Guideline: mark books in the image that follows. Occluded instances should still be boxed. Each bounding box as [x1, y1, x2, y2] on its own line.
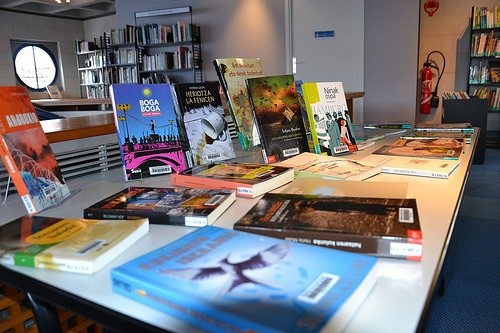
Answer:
[471, 30, 500, 58]
[0, 57, 474, 216]
[143, 45, 199, 71]
[472, 87, 500, 110]
[235, 193, 424, 261]
[76, 38, 100, 53]
[470, 59, 500, 83]
[136, 21, 196, 45]
[91, 50, 111, 66]
[111, 224, 381, 333]
[109, 24, 136, 45]
[472, 6, 500, 30]
[112, 46, 145, 64]
[0, 214, 149, 274]
[84, 186, 236, 228]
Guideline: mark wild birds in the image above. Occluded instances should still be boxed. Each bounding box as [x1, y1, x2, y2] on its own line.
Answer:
[156, 241, 293, 303]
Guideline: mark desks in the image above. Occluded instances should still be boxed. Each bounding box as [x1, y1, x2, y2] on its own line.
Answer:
[0, 126, 481, 333]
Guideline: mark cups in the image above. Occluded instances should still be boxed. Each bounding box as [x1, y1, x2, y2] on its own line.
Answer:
[201, 110, 227, 141]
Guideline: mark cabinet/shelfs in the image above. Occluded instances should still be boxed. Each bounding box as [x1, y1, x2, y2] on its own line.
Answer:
[466, 6, 500, 113]
[74, 24, 203, 112]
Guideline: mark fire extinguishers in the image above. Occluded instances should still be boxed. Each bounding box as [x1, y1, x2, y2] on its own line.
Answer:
[420, 50, 446, 114]
